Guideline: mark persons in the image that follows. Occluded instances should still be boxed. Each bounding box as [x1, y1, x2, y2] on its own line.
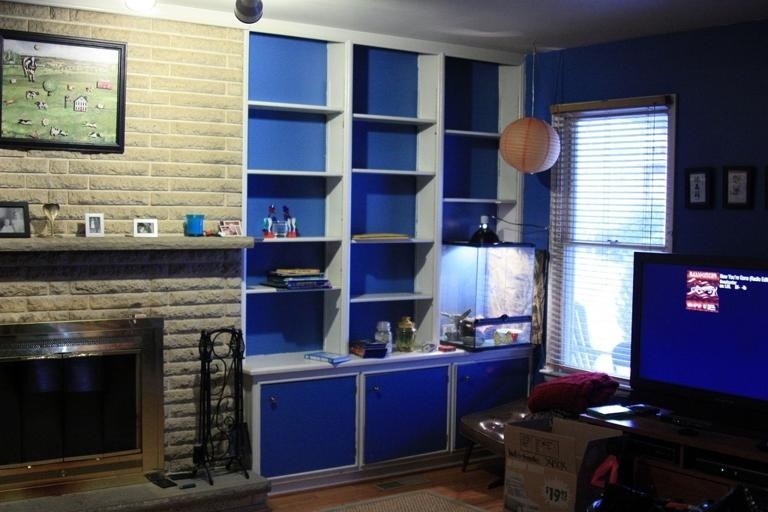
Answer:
[2, 219, 14, 233]
[222, 221, 241, 235]
[90, 217, 101, 233]
[138, 222, 151, 233]
[12, 211, 24, 232]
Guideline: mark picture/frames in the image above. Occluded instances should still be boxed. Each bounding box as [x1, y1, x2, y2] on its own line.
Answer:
[683, 166, 714, 210]
[2, 200, 32, 239]
[132, 218, 161, 239]
[0, 29, 128, 155]
[84, 211, 105, 239]
[721, 165, 755, 210]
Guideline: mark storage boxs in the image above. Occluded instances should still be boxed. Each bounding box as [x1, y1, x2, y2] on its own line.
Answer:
[502, 416, 624, 512]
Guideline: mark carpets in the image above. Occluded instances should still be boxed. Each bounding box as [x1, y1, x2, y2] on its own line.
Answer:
[317, 490, 486, 512]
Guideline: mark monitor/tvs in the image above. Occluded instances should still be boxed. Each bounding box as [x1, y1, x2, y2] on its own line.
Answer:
[630, 252, 768, 435]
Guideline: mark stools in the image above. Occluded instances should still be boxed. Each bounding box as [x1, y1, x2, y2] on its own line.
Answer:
[458, 391, 535, 473]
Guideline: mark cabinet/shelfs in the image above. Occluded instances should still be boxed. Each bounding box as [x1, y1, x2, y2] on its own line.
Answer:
[242, 373, 362, 497]
[361, 361, 452, 483]
[441, 45, 524, 248]
[451, 349, 535, 466]
[347, 36, 443, 355]
[244, 17, 347, 359]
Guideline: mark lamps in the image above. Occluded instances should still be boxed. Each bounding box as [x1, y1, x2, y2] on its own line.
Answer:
[469, 211, 549, 247]
[497, 39, 563, 178]
[233, 0, 266, 24]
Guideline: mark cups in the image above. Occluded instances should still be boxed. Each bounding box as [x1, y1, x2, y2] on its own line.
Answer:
[185, 212, 205, 236]
[271, 220, 289, 237]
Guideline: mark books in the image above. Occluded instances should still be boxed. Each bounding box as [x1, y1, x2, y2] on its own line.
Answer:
[351, 232, 408, 238]
[259, 268, 332, 290]
[303, 352, 350, 364]
[352, 236, 409, 241]
[349, 338, 386, 358]
[587, 404, 632, 420]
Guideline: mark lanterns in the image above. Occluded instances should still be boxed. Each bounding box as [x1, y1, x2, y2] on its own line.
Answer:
[498, 115, 562, 175]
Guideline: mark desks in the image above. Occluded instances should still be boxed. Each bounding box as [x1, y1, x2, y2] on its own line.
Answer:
[577, 412, 768, 506]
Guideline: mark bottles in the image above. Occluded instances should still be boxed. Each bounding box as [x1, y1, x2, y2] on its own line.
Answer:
[375, 320, 392, 353]
[396, 314, 415, 352]
